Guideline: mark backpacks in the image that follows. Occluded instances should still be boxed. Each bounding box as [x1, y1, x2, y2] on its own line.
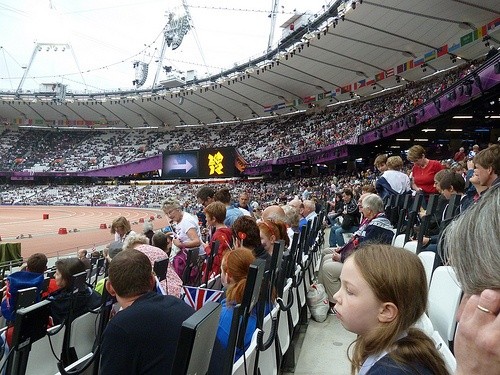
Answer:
[173, 241, 200, 283]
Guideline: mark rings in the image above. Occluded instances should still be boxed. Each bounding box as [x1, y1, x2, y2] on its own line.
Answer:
[477, 305, 496, 315]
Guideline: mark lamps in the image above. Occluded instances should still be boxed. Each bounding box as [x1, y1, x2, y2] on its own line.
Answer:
[449, 54, 457, 64]
[482, 35, 492, 49]
[2, 0, 377, 131]
[395, 75, 401, 83]
[420, 63, 428, 73]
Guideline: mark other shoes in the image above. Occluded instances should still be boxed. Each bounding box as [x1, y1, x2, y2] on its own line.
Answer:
[327, 306, 337, 315]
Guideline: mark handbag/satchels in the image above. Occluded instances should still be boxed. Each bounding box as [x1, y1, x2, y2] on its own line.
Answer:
[342, 215, 354, 230]
[309, 282, 329, 322]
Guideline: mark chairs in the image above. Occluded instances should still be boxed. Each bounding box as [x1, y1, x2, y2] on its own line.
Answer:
[0, 190, 500, 375]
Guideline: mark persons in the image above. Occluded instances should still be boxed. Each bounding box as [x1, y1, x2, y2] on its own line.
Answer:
[444, 182, 500, 375]
[333, 244, 449, 375]
[0, 47, 500, 375]
[213, 248, 274, 375]
[99, 249, 196, 375]
